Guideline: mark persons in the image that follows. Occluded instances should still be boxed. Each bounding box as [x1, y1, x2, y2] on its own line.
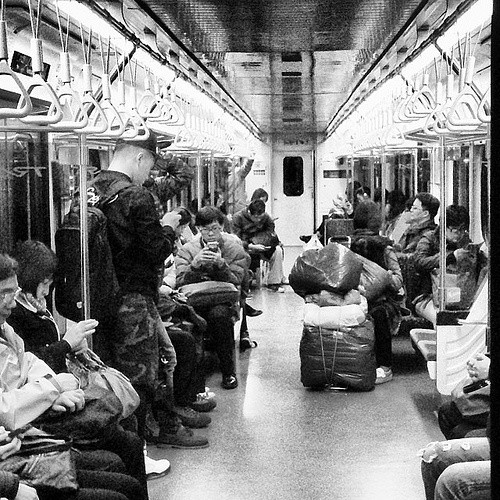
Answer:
[159, 152, 288, 425]
[420, 354, 491, 500]
[299, 180, 479, 385]
[0, 239, 148, 500]
[70, 124, 208, 479]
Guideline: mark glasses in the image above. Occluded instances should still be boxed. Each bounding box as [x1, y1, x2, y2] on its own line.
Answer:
[1, 286, 23, 303]
[199, 224, 218, 236]
[448, 228, 468, 234]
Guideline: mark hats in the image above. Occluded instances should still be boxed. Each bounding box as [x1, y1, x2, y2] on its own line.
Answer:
[173, 206, 192, 225]
[116, 127, 161, 161]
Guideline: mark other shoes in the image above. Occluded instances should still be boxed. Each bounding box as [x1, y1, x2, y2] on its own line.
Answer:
[299, 236, 311, 243]
[172, 406, 213, 428]
[156, 417, 210, 449]
[374, 368, 393, 385]
[188, 394, 217, 413]
[241, 331, 252, 348]
[145, 455, 171, 481]
[221, 366, 238, 389]
[266, 284, 286, 292]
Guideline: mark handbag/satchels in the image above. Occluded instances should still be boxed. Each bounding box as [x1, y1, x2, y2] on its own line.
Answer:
[32, 385, 124, 439]
[451, 374, 490, 416]
[0, 423, 79, 495]
[178, 280, 239, 310]
[430, 255, 477, 309]
[74, 350, 141, 422]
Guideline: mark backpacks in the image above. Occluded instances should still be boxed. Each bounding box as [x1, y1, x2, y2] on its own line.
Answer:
[54, 180, 138, 332]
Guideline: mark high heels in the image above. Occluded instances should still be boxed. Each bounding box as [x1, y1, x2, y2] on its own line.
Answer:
[246, 309, 263, 316]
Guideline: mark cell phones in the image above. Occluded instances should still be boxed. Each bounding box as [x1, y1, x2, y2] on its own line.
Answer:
[207, 242, 219, 253]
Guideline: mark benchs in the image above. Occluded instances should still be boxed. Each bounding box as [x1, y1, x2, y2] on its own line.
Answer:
[409, 271, 490, 363]
